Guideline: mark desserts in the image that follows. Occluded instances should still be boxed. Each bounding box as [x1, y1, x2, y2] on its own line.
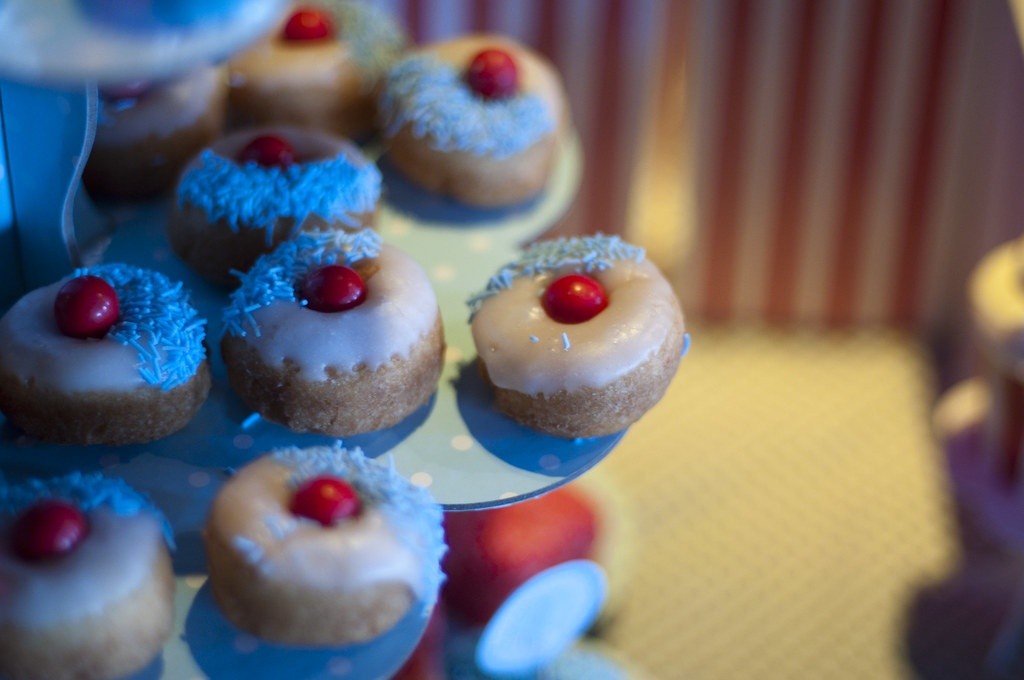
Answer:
[0, 0, 685, 680]
